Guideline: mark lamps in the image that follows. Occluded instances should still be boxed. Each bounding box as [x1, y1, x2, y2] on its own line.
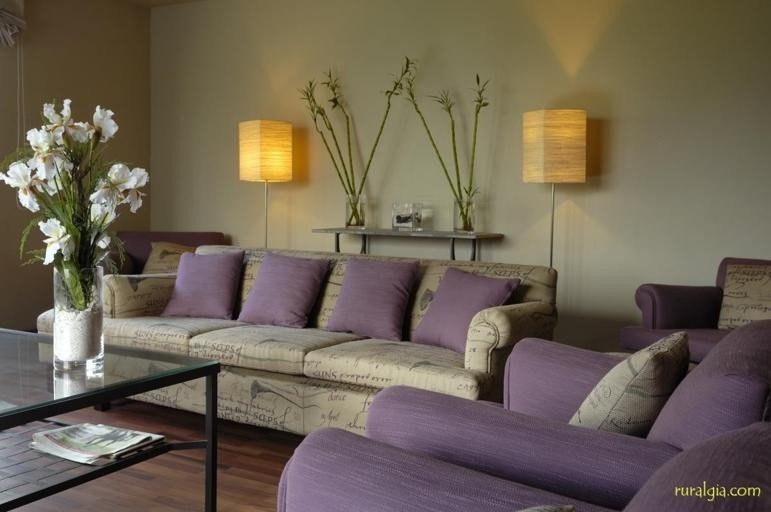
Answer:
[238, 119, 292, 248]
[521, 109, 588, 268]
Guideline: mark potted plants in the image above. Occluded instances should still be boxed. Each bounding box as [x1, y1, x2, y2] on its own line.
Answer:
[295, 55, 415, 230]
[400, 70, 491, 233]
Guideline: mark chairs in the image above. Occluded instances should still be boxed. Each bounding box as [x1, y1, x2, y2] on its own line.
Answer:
[97, 231, 223, 275]
[618, 257, 771, 364]
[365, 318, 771, 512]
[276, 422, 771, 512]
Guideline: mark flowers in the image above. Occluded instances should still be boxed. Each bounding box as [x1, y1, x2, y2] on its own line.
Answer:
[0, 97, 150, 310]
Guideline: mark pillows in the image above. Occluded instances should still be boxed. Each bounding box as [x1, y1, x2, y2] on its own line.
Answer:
[160, 250, 246, 320]
[568, 330, 690, 436]
[142, 241, 197, 275]
[238, 251, 337, 328]
[324, 258, 420, 341]
[413, 267, 521, 356]
[717, 264, 771, 330]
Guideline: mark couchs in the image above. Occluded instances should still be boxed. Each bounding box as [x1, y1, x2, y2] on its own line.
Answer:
[37, 244, 557, 436]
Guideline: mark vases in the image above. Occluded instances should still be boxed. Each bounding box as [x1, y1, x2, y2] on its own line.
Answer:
[53, 266, 104, 370]
[52, 369, 104, 399]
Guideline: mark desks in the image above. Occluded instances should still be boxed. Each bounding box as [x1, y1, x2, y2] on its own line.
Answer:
[311, 227, 505, 262]
